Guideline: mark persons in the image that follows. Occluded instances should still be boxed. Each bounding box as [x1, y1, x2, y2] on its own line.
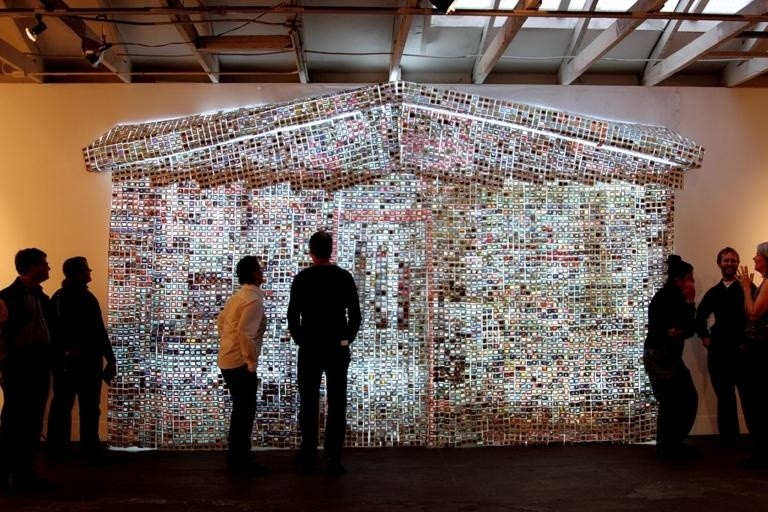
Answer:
[288, 231, 361, 478]
[0, 249, 53, 496]
[218, 257, 267, 475]
[644, 242, 768, 468]
[43, 257, 116, 466]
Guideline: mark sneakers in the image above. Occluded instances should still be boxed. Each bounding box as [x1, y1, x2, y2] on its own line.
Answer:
[294, 457, 348, 478]
[225, 447, 266, 472]
[43, 439, 118, 461]
[719, 433, 768, 468]
[1, 471, 60, 498]
[654, 441, 703, 461]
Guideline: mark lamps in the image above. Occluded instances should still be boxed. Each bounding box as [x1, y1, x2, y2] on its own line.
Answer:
[22, 13, 48, 42]
[79, 35, 112, 67]
